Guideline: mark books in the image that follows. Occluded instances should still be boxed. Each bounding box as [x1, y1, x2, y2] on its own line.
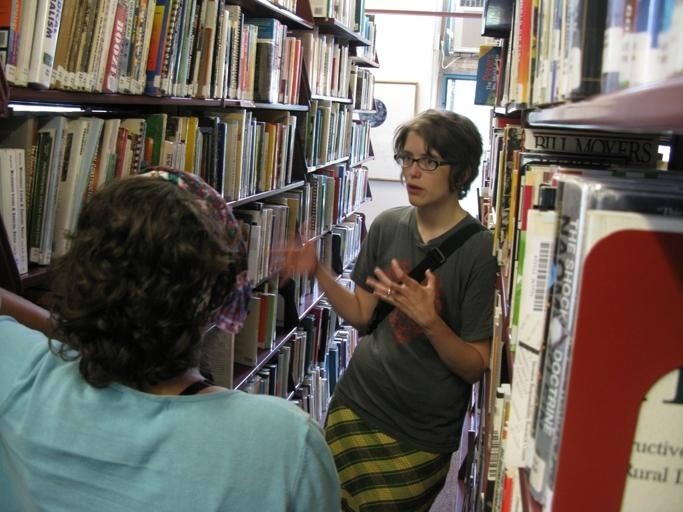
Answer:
[465, 2, 682, 511]
[1, 1, 375, 422]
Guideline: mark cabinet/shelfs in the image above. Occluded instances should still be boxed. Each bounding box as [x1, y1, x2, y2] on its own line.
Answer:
[470, 1, 682, 512]
[0, 1, 377, 429]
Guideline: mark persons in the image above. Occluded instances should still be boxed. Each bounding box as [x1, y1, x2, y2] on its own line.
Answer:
[0, 167, 341, 512]
[269, 108, 497, 512]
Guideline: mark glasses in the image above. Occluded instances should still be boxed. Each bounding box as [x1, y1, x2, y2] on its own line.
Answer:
[394, 153, 452, 171]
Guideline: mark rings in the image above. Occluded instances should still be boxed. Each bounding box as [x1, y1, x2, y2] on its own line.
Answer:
[384, 287, 392, 296]
